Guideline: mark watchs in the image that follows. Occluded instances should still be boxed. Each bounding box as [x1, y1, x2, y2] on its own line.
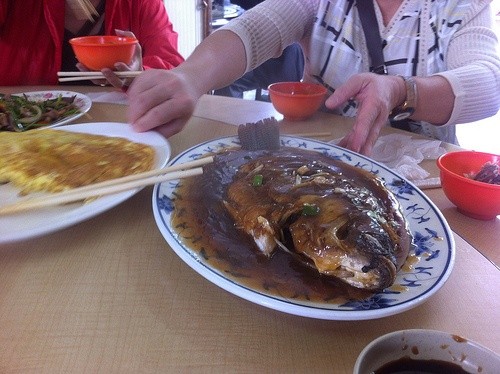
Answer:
[388, 74, 418, 121]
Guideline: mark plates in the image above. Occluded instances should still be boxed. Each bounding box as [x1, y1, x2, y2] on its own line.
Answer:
[11, 89, 92, 130]
[0, 122, 170, 244]
[151, 138, 457, 321]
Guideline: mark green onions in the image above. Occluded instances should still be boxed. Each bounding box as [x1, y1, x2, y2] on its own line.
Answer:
[252, 165, 350, 215]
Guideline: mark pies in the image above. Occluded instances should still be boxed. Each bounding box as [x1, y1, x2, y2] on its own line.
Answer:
[0, 130, 157, 205]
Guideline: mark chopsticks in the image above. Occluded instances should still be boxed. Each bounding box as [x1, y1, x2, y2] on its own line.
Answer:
[57, 71, 146, 82]
[0, 154, 213, 216]
[78, 0, 99, 24]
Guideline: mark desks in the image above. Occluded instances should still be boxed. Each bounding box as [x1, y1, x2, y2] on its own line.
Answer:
[0, 85, 500, 374]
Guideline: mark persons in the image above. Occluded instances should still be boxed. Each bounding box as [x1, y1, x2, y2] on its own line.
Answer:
[126, 0, 500, 140]
[214, 0, 305, 98]
[0, 0, 185, 88]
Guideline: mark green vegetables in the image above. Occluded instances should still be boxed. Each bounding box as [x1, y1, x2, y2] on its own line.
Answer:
[0, 95, 80, 132]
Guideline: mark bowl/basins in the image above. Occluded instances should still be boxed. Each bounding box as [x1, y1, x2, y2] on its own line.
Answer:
[70, 35, 138, 72]
[267, 82, 328, 121]
[436, 150, 500, 221]
[352, 328, 500, 374]
